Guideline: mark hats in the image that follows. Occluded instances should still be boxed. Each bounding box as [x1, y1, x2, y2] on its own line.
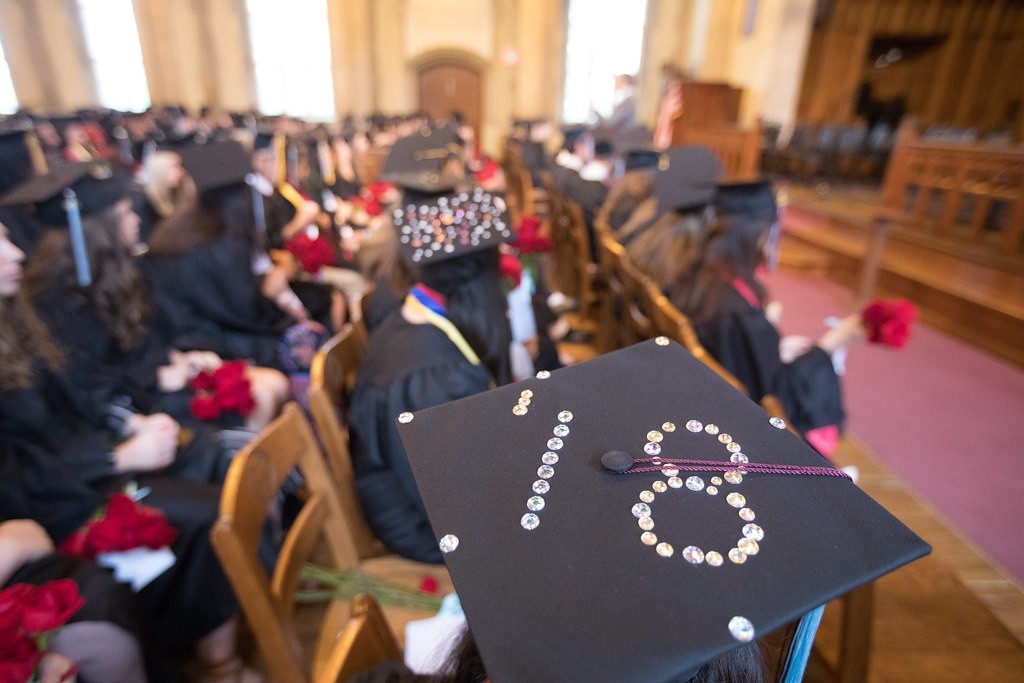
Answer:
[393, 335, 932, 683]
[0, 92, 788, 280]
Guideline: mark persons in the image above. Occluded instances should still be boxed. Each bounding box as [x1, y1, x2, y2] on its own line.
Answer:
[514, 73, 868, 486]
[0, 103, 567, 683]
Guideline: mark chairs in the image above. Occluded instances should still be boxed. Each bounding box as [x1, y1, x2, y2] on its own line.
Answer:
[503, 145, 749, 398]
[208, 293, 456, 683]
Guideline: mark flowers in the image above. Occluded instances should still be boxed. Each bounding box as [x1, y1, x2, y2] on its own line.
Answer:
[351, 181, 390, 217]
[499, 251, 523, 292]
[514, 216, 555, 255]
[191, 358, 255, 420]
[477, 155, 500, 184]
[287, 232, 336, 274]
[63, 489, 180, 556]
[0, 579, 85, 683]
[854, 298, 919, 350]
